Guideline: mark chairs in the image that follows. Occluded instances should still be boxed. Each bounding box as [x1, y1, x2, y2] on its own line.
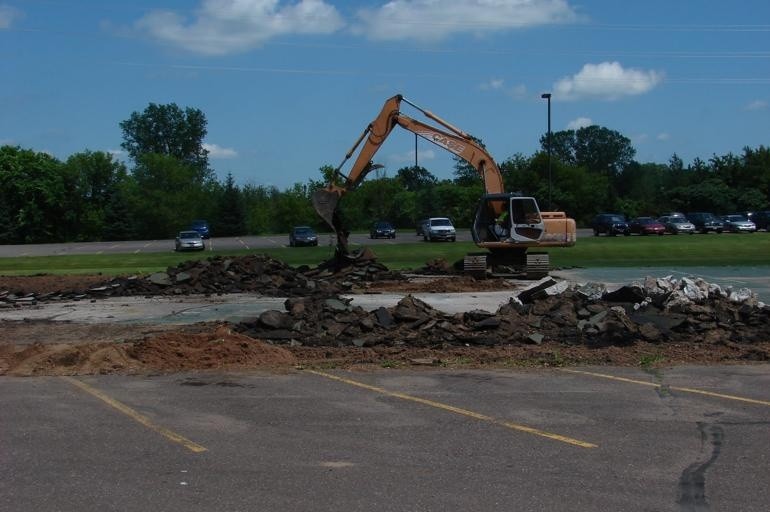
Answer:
[493, 204, 511, 242]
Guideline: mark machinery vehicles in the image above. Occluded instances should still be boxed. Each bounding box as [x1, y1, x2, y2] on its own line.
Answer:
[311, 93, 578, 280]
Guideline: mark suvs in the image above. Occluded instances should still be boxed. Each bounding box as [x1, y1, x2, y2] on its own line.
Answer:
[750, 207, 770, 232]
[686, 212, 725, 234]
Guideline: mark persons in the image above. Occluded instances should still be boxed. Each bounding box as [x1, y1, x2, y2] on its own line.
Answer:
[489, 202, 509, 241]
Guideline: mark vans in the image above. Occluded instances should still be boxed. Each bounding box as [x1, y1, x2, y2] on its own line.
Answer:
[191, 219, 211, 239]
[422, 217, 456, 242]
[593, 212, 633, 237]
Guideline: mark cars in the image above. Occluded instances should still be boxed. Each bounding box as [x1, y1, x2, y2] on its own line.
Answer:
[416, 218, 429, 235]
[657, 215, 696, 235]
[175, 230, 206, 252]
[663, 213, 688, 222]
[369, 220, 396, 239]
[289, 226, 319, 247]
[722, 214, 757, 233]
[628, 216, 666, 237]
